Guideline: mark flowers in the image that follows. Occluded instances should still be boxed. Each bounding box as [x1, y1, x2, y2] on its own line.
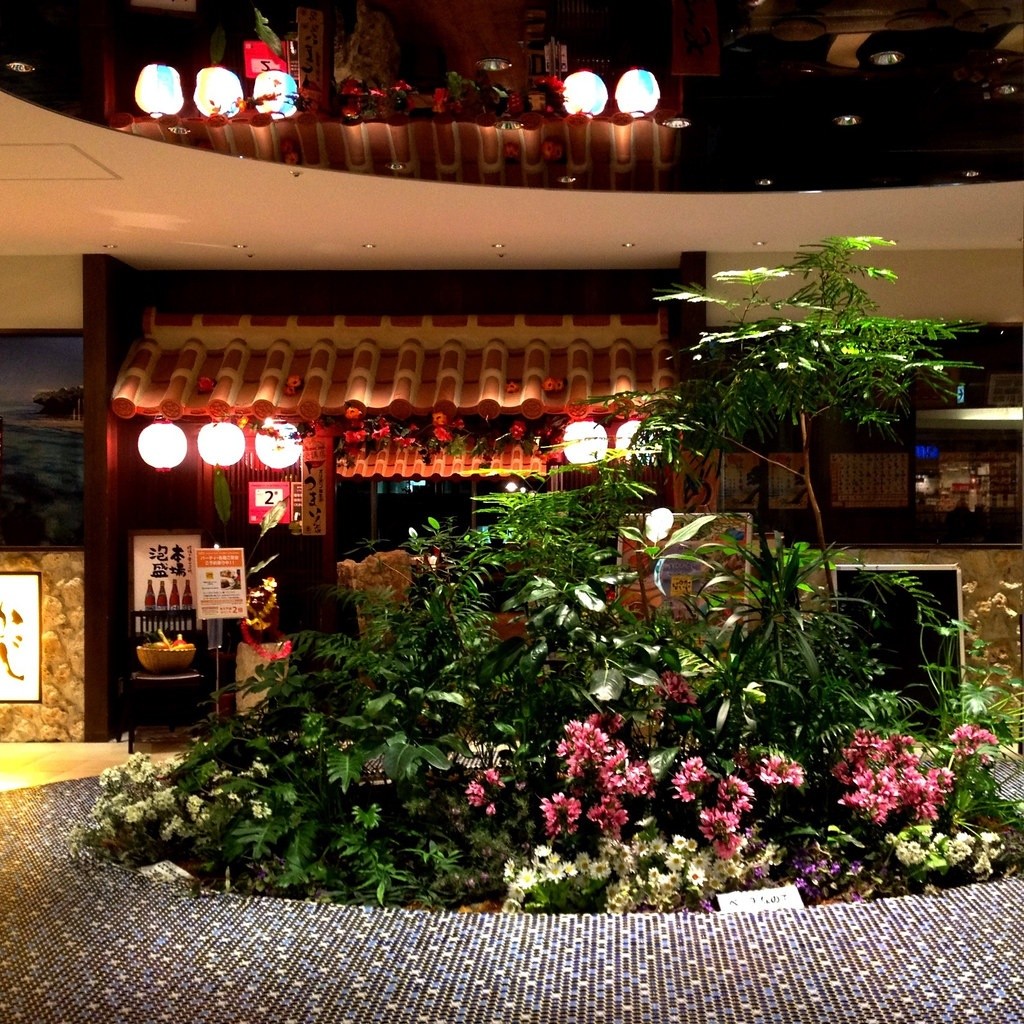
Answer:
[312, 407, 544, 462]
[332, 73, 568, 119]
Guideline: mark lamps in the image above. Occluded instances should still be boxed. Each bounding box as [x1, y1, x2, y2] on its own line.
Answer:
[197, 422, 246, 471]
[192, 66, 244, 121]
[561, 71, 608, 119]
[255, 422, 302, 474]
[252, 70, 300, 121]
[560, 420, 608, 465]
[618, 69, 661, 119]
[617, 419, 657, 466]
[136, 414, 188, 474]
[134, 63, 184, 119]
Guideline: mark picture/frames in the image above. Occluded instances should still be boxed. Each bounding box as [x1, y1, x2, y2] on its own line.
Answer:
[830, 562, 966, 744]
[610, 512, 753, 642]
[0, 328, 84, 551]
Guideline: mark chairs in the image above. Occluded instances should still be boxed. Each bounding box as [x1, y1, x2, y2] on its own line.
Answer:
[115, 609, 207, 755]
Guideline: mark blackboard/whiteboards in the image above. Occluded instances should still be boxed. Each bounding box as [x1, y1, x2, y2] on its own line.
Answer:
[828, 562, 966, 739]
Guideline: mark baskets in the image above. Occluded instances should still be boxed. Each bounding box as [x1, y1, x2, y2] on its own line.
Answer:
[137, 645, 196, 674]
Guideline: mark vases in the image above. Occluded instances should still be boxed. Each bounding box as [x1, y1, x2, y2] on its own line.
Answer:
[528, 91, 547, 110]
